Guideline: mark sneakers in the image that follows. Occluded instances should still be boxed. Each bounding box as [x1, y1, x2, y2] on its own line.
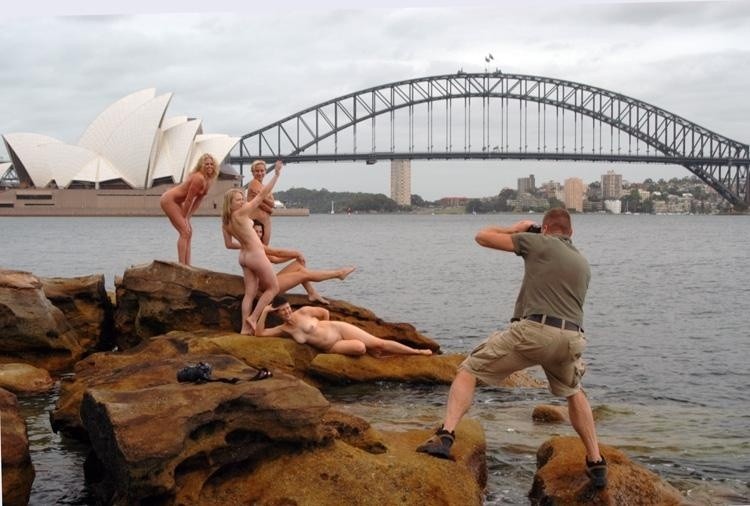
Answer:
[585, 454, 608, 488]
[415, 424, 455, 456]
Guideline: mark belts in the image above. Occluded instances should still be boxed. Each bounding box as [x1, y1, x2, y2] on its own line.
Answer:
[510, 313, 586, 335]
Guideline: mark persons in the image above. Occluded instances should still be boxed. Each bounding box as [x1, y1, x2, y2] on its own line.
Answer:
[251, 219, 356, 305]
[160, 153, 219, 265]
[247, 160, 276, 246]
[221, 160, 282, 335]
[416, 210, 608, 487]
[254, 296, 433, 358]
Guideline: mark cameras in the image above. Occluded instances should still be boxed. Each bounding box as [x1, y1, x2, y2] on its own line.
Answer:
[177, 363, 209, 384]
[527, 224, 543, 235]
